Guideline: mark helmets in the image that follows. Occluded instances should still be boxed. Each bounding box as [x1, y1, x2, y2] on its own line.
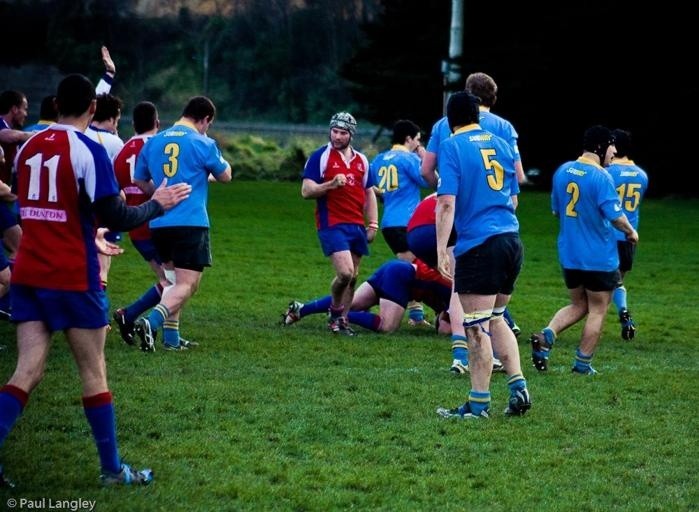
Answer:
[583, 125, 617, 167]
[447, 91, 479, 134]
[585, 127, 615, 167]
[329, 111, 358, 142]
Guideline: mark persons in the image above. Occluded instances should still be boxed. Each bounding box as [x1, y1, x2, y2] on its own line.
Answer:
[1, 72, 154, 491]
[602, 126, 651, 342]
[530, 120, 639, 378]
[282, 71, 532, 421]
[1, 44, 234, 354]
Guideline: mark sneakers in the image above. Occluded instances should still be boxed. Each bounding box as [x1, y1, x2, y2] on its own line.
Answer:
[282, 301, 635, 415]
[112, 306, 200, 353]
[99, 464, 155, 498]
